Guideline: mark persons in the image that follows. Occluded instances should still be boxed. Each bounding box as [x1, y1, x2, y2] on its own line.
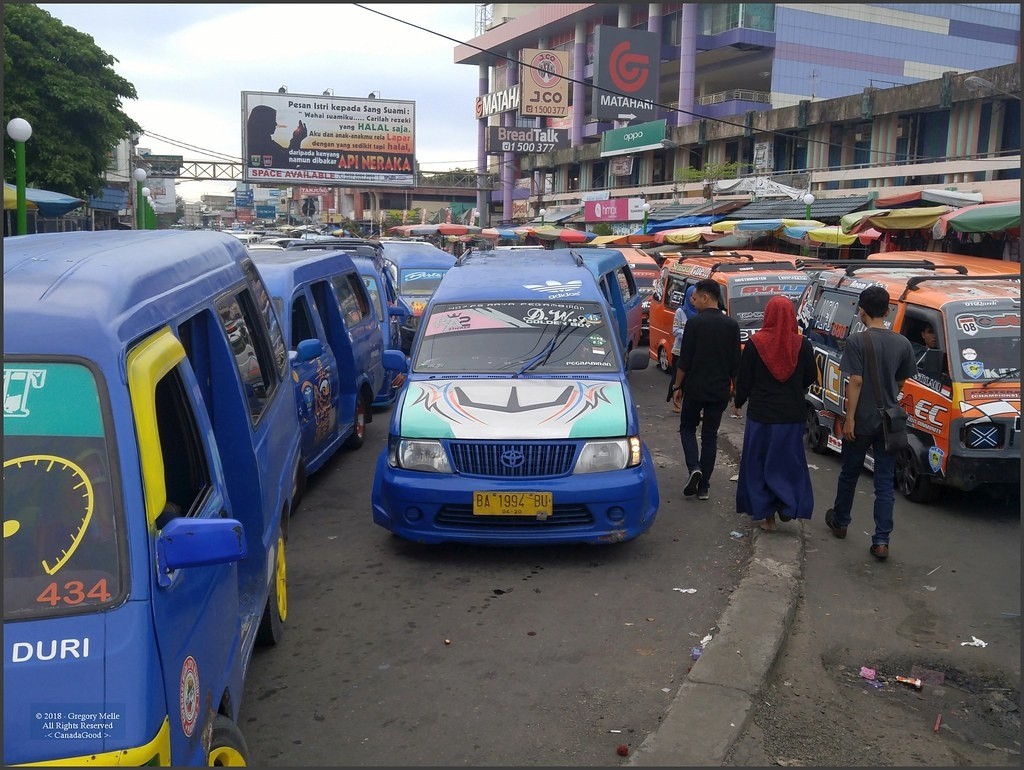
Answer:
[823, 285, 918, 558]
[734, 296, 818, 531]
[912, 323, 951, 368]
[667, 278, 742, 500]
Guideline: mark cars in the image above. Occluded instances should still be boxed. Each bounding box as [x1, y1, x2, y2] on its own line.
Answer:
[373, 248, 660, 548]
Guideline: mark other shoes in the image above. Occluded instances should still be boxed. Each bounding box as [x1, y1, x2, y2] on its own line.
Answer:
[673, 407, 680, 412]
[826, 509, 848, 538]
[870, 545, 888, 557]
[760, 519, 776, 530]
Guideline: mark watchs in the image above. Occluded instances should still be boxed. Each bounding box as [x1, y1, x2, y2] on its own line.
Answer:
[672, 385, 681, 390]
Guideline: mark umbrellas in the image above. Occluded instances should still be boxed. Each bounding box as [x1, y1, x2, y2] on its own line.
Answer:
[389, 220, 481, 253]
[483, 216, 596, 252]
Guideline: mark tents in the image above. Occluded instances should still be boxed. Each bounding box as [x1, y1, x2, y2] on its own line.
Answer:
[2, 184, 87, 237]
[570, 212, 863, 261]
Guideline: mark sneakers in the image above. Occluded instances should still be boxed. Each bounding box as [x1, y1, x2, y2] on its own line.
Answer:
[697, 483, 710, 499]
[683, 468, 702, 496]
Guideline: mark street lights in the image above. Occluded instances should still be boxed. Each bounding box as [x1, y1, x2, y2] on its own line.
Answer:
[804, 194, 815, 221]
[643, 202, 651, 235]
[539, 208, 547, 227]
[474, 212, 482, 228]
[134, 166, 147, 230]
[7, 117, 32, 234]
[141, 187, 150, 229]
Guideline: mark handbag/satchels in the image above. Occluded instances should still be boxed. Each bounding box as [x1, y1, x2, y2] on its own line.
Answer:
[878, 407, 909, 452]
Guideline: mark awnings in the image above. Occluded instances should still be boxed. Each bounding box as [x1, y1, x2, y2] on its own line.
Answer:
[640, 189, 1022, 218]
[531, 208, 585, 223]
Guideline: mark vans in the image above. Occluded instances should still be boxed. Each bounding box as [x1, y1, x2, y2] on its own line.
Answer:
[3, 227, 303, 769]
[784, 256, 1021, 505]
[211, 250, 386, 521]
[222, 219, 1021, 380]
